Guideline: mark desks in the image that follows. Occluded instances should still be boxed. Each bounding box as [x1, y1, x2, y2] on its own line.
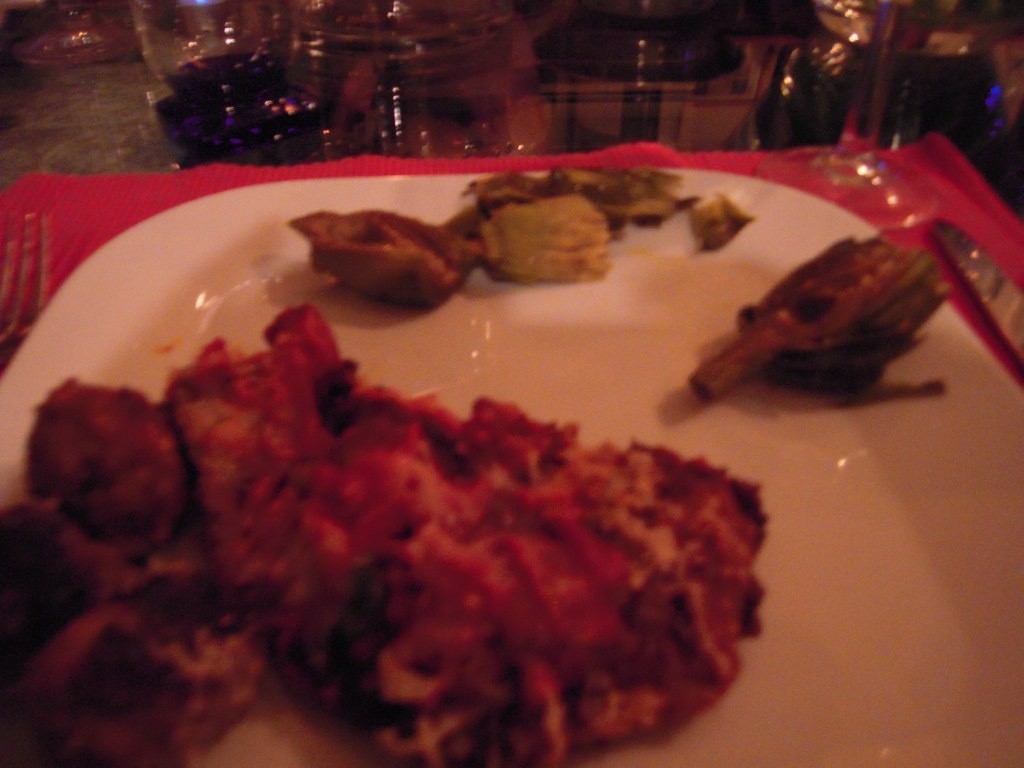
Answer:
[1, 137, 1024, 419]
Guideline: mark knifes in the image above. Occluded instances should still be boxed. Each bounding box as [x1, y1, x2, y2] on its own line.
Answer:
[933, 216, 1024, 366]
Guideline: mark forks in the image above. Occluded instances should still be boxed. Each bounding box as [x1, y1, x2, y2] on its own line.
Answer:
[0, 214, 48, 358]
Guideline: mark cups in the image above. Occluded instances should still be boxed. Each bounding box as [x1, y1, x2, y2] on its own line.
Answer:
[1, 0, 1024, 165]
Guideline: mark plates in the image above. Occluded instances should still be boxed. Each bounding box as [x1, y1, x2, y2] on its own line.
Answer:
[3, 170, 1024, 767]
